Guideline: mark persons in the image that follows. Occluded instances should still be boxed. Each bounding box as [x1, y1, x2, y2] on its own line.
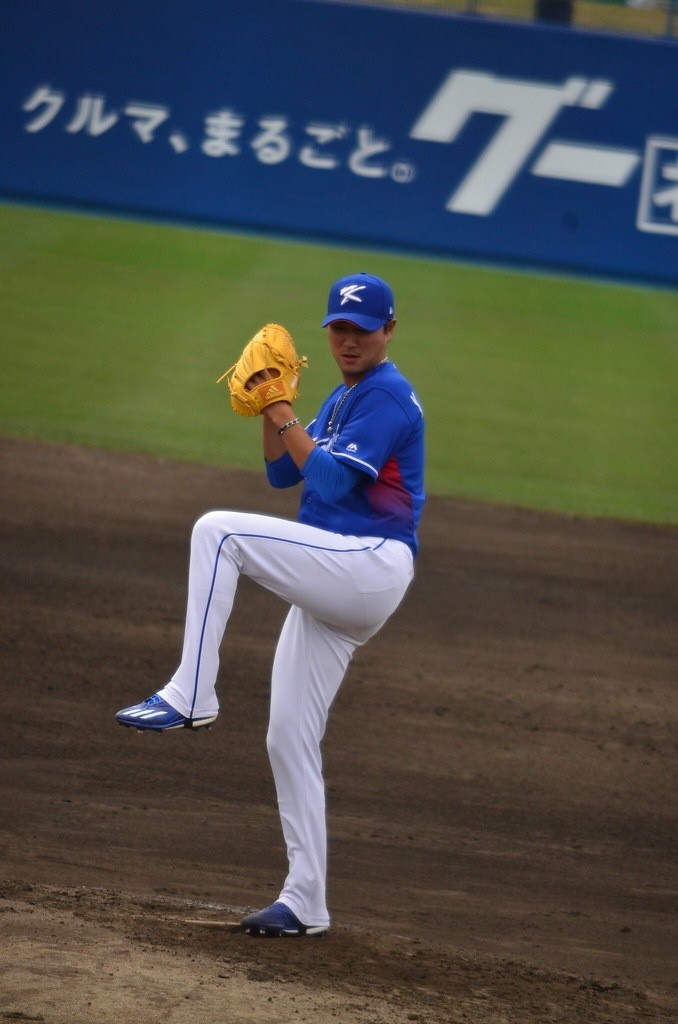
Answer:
[113, 272, 427, 940]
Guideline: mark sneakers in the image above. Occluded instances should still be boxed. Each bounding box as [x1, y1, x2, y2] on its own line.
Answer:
[114, 685, 217, 736]
[240, 902, 329, 939]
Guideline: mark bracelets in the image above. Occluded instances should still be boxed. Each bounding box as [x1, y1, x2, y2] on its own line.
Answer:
[276, 417, 302, 437]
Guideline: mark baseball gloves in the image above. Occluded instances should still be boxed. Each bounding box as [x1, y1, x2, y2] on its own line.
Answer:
[230, 323, 303, 418]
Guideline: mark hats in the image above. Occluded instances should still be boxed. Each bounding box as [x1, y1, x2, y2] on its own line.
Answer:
[321, 271, 395, 331]
